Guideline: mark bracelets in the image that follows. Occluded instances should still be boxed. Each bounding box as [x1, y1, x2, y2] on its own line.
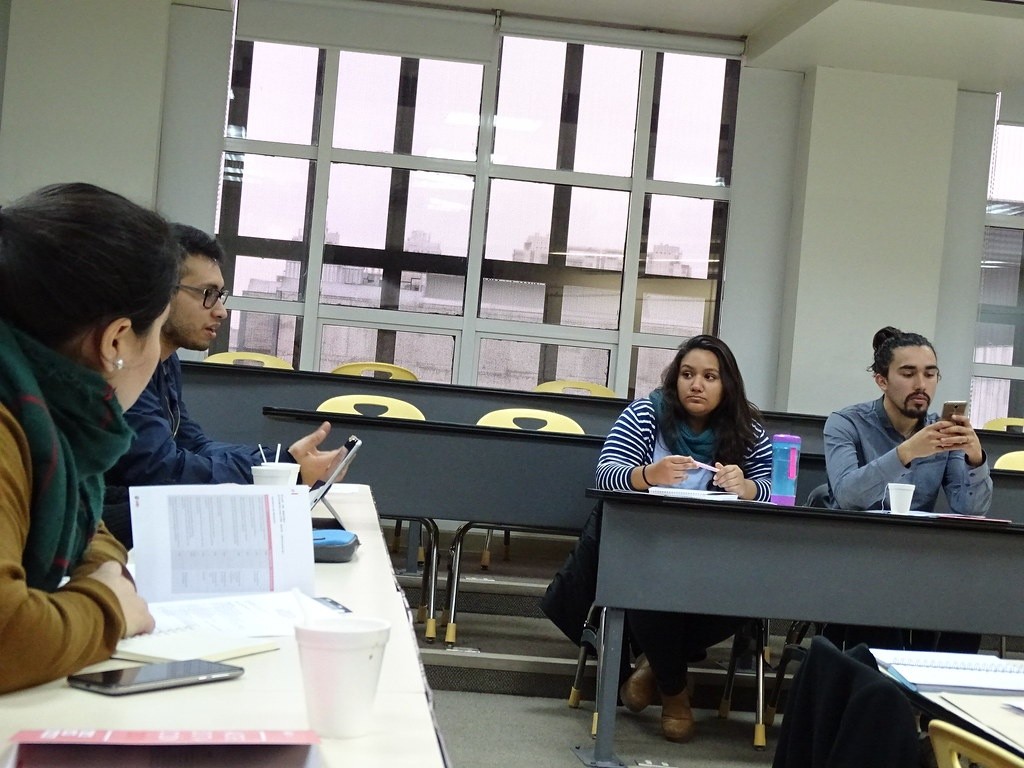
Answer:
[642, 465, 658, 486]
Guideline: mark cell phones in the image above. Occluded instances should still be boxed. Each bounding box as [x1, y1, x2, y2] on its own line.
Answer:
[309, 435, 362, 508]
[942, 401, 967, 434]
[68, 660, 244, 696]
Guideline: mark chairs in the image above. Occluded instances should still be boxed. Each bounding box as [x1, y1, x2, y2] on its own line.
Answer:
[772, 635, 917, 768]
[983, 418, 1024, 431]
[203, 352, 295, 370]
[331, 362, 425, 565]
[316, 395, 440, 644]
[993, 450, 1024, 471]
[480, 380, 616, 568]
[928, 718, 1024, 768]
[446, 408, 585, 645]
[764, 484, 1007, 725]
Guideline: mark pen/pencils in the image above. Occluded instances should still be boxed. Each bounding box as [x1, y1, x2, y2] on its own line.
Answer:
[695, 461, 720, 473]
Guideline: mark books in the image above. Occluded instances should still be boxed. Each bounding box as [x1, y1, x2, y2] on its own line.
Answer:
[869, 648, 1024, 715]
[649, 487, 738, 500]
[111, 485, 340, 662]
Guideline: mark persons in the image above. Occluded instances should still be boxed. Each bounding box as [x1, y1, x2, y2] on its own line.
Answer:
[0, 181, 190, 693]
[595, 334, 773, 743]
[822, 324, 993, 669]
[102, 222, 349, 550]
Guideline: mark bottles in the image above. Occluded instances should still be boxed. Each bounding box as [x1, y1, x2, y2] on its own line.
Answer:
[772, 434, 800, 505]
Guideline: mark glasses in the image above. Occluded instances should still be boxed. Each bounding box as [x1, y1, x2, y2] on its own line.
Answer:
[175, 284, 229, 309]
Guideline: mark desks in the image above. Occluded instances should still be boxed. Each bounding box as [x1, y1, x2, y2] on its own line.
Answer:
[0, 359, 1024, 768]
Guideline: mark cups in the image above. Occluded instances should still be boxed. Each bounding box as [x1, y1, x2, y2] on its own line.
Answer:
[887, 482, 916, 514]
[295, 618, 393, 741]
[263, 463, 300, 485]
[252, 465, 289, 490]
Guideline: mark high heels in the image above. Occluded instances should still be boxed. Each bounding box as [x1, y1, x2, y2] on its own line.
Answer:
[620, 652, 658, 712]
[662, 677, 694, 743]
